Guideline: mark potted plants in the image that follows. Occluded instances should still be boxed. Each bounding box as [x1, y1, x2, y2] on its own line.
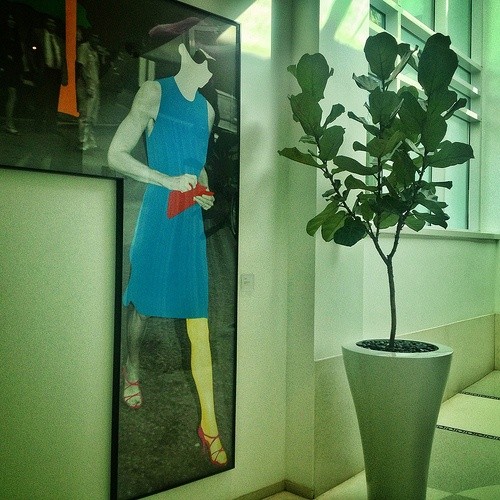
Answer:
[277, 32, 477, 500]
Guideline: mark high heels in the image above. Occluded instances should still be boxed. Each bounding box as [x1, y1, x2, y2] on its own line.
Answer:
[122, 364, 142, 410]
[196, 424, 230, 470]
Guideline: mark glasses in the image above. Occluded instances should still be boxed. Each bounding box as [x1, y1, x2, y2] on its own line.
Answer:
[190, 45, 218, 75]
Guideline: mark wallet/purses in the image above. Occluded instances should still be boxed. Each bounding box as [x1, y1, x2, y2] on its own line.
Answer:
[168, 181, 206, 217]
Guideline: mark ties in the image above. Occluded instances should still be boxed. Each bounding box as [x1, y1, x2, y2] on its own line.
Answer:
[48, 35, 58, 72]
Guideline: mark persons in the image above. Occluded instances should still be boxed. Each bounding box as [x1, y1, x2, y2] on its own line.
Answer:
[105, 20, 230, 468]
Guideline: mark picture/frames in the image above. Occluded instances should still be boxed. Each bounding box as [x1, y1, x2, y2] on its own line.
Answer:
[0, 0, 243, 500]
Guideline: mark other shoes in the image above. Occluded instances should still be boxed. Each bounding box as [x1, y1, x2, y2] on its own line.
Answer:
[5, 125, 18, 135]
[79, 140, 98, 151]
